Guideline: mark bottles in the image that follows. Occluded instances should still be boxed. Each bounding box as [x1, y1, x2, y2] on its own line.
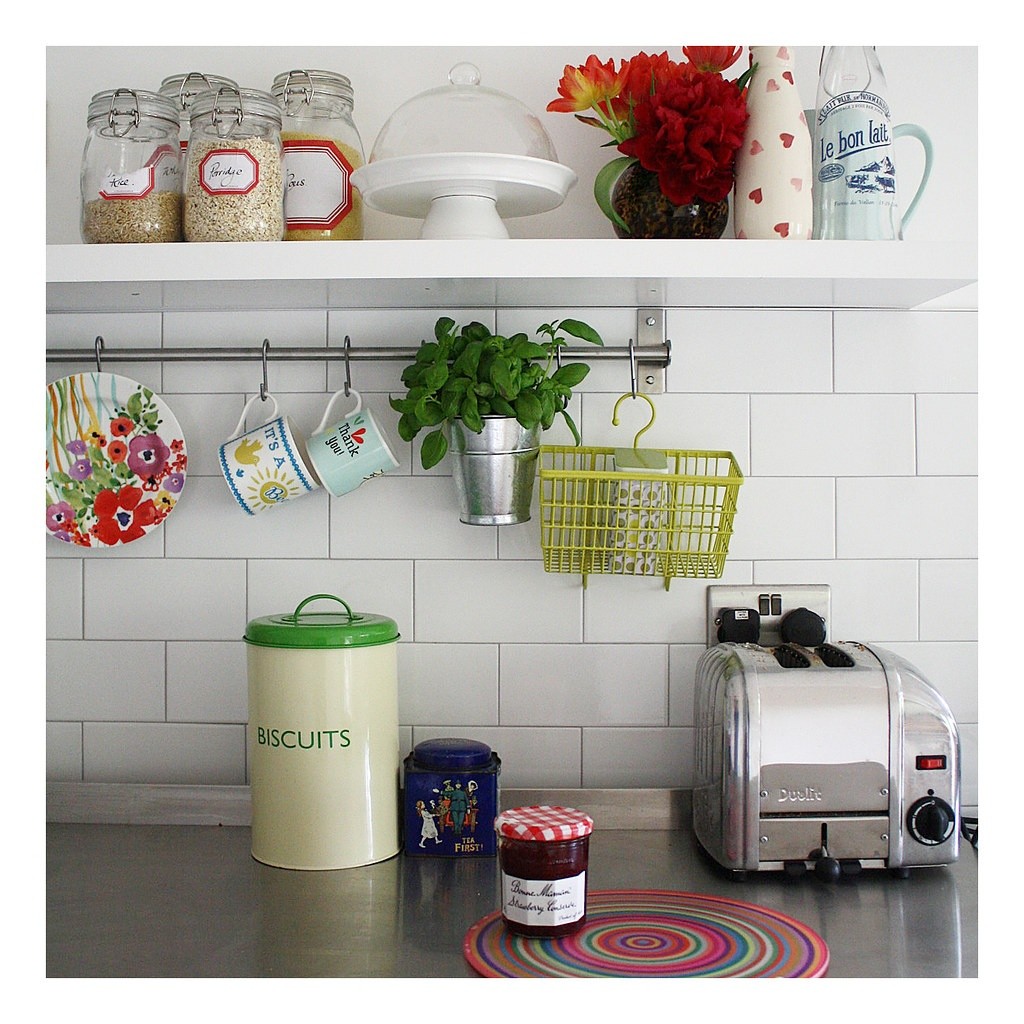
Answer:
[181, 84, 288, 243]
[156, 72, 238, 174]
[732, 46, 817, 238]
[808, 46, 933, 241]
[270, 69, 368, 242]
[78, 90, 186, 244]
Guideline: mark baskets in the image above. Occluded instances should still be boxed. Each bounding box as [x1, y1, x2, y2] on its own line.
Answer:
[540, 393, 743, 592]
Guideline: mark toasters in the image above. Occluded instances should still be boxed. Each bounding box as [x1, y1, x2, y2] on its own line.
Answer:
[694, 636, 964, 878]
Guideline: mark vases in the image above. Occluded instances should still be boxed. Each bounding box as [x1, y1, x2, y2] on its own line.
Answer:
[593, 156, 730, 240]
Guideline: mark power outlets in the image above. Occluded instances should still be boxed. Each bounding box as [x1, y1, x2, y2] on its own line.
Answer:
[707, 583, 832, 648]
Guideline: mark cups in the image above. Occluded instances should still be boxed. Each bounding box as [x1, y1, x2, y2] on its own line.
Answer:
[305, 389, 401, 497]
[218, 393, 320, 516]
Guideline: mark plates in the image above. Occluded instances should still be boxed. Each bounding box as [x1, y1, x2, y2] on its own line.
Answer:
[46, 371, 187, 549]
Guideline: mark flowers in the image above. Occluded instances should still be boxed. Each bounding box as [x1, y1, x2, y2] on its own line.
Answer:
[547, 45, 757, 206]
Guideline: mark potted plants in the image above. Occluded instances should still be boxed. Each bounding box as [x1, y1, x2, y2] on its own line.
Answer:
[387, 316, 604, 526]
